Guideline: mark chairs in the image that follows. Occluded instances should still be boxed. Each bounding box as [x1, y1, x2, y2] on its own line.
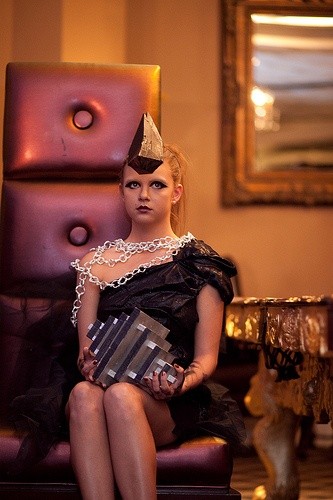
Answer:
[0, 60, 240, 500]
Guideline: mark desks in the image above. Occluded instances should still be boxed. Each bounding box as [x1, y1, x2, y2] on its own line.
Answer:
[227, 295, 333, 499]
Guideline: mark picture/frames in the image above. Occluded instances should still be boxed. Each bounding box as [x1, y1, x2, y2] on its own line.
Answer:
[218, 0, 333, 209]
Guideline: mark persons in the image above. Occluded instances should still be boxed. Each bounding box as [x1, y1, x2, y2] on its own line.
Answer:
[6, 109, 247, 500]
